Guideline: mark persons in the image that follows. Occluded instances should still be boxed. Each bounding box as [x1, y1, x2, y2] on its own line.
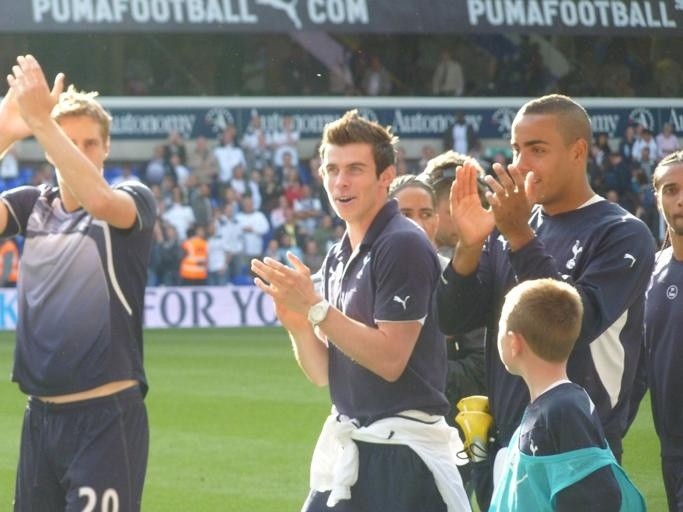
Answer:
[0, 53, 157, 512]
[420, 149, 496, 512]
[489, 277, 646, 512]
[588, 117, 683, 249]
[113, 123, 343, 287]
[250, 109, 474, 512]
[430, 93, 656, 512]
[619, 149, 683, 512]
[385, 174, 493, 512]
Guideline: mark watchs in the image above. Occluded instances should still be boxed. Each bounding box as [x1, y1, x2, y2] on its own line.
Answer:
[307, 298, 330, 327]
